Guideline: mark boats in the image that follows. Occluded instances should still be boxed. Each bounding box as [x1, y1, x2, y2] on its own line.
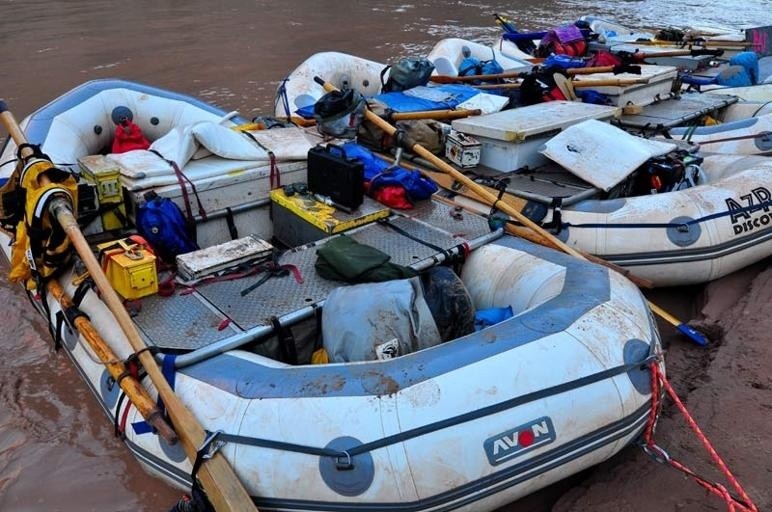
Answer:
[422, 15, 772, 157]
[0, 79, 666, 511]
[273, 51, 772, 287]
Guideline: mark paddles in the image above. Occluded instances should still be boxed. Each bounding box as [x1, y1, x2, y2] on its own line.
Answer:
[624, 26, 772, 55]
[229, 109, 480, 132]
[0, 99, 258, 510]
[373, 151, 547, 223]
[427, 66, 614, 83]
[315, 76, 709, 346]
[553, 71, 578, 102]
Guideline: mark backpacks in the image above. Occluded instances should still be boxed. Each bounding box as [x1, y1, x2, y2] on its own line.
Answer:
[132, 189, 201, 268]
[312, 86, 369, 141]
[378, 56, 436, 95]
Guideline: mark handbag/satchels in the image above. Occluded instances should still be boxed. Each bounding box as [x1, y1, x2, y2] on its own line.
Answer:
[456, 56, 507, 90]
[537, 20, 594, 59]
